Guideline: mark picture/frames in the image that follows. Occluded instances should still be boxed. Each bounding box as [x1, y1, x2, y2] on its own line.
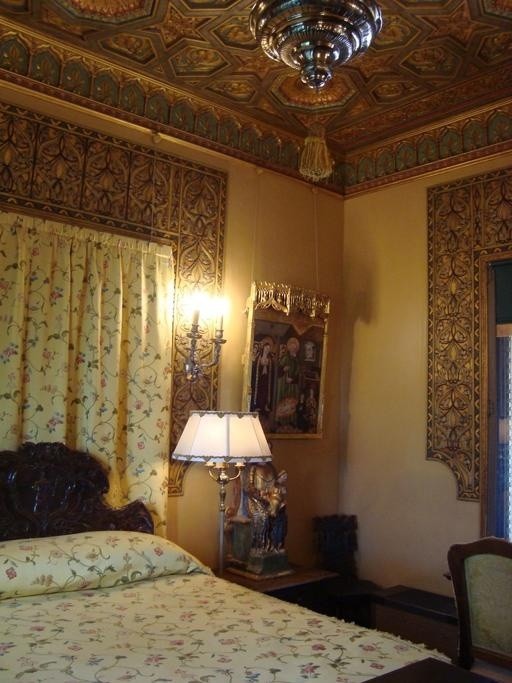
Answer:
[241, 280, 332, 440]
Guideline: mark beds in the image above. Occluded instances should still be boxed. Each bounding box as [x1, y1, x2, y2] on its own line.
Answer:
[0, 441, 497, 683]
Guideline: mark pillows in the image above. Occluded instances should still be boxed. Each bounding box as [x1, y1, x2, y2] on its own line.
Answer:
[0, 531, 216, 600]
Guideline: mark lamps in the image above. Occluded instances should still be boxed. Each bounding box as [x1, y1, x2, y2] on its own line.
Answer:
[172, 410, 273, 577]
[248, 0, 384, 178]
[172, 293, 228, 383]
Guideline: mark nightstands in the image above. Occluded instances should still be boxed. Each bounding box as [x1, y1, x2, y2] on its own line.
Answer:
[211, 562, 340, 617]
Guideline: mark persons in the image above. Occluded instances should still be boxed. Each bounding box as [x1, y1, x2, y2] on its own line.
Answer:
[251, 336, 321, 434]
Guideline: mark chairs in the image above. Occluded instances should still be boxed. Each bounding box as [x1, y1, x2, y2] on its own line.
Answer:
[448, 536, 512, 683]
